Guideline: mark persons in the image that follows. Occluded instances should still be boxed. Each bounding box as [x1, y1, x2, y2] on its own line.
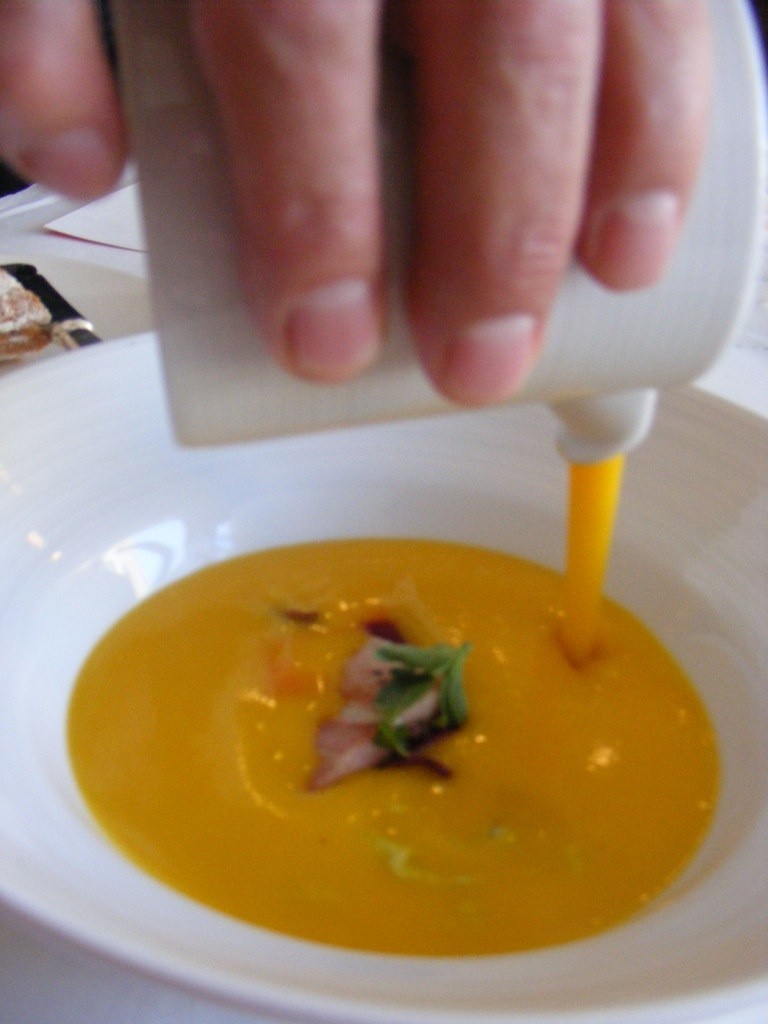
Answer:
[0, 0, 701, 406]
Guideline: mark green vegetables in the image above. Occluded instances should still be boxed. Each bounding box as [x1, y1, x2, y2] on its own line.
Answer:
[369, 637, 473, 757]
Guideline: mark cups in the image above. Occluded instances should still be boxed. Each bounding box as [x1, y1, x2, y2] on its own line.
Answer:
[109, 0, 768, 450]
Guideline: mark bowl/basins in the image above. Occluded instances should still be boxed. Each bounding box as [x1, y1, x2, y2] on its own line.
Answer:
[0, 325, 767, 1024]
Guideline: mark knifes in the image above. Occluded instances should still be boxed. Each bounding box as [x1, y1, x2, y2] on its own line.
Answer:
[2, 260, 104, 353]
